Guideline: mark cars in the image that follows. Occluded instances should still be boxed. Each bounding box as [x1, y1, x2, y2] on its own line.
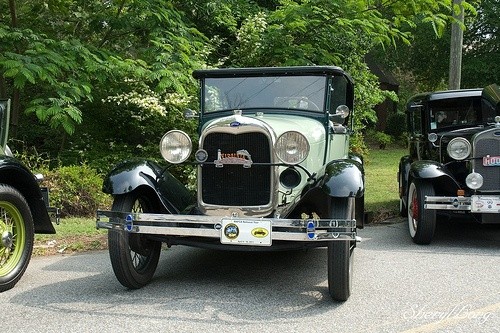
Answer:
[0, 96, 61, 292]
[397, 87, 500, 246]
[96, 65, 372, 303]
